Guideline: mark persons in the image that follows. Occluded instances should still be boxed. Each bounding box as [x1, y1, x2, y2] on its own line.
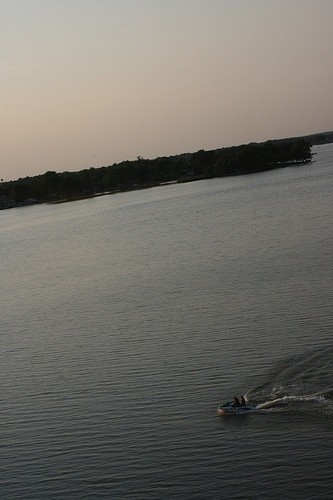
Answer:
[233, 396, 239, 406]
[240, 395, 245, 404]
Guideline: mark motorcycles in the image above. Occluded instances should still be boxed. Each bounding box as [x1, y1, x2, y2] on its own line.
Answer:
[219, 401, 252, 412]
